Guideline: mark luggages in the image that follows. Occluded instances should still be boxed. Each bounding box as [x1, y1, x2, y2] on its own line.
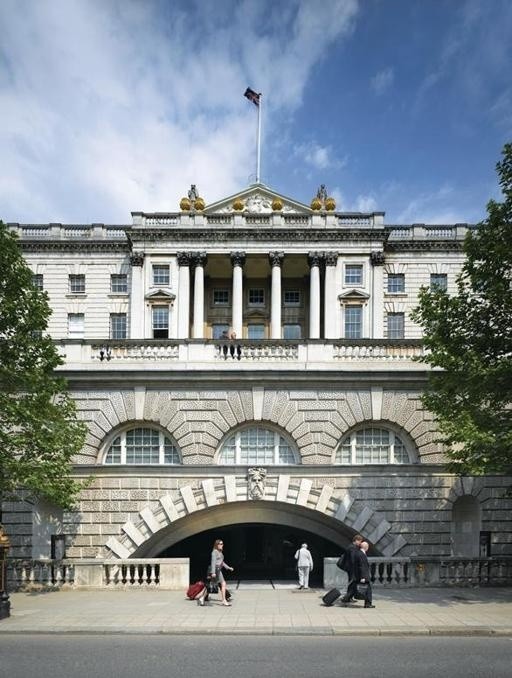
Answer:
[187, 581, 205, 600]
[323, 580, 353, 605]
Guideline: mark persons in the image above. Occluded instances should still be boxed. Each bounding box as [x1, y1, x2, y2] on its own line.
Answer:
[218, 329, 229, 357]
[188, 184, 198, 210]
[317, 184, 328, 209]
[229, 332, 241, 360]
[343, 533, 363, 602]
[197, 538, 234, 606]
[247, 467, 267, 498]
[294, 542, 314, 588]
[343, 541, 375, 607]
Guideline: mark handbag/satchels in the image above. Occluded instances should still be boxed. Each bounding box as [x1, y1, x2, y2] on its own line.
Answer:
[337, 555, 351, 571]
[354, 584, 369, 599]
[207, 578, 218, 593]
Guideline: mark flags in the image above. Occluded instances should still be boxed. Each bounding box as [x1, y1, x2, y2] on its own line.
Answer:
[243, 86, 259, 107]
